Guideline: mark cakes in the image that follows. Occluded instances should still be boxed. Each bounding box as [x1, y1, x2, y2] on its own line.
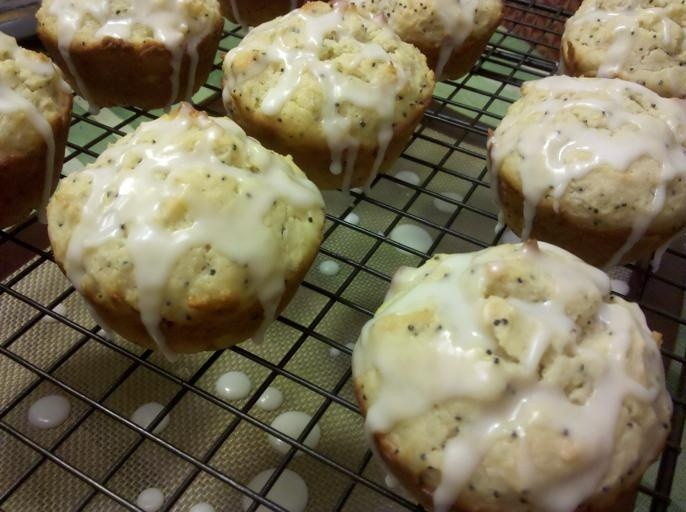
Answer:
[356, 0, 505, 80]
[35, 0, 224, 110]
[486, 75, 686, 270]
[1, 32, 74, 229]
[222, 1, 436, 190]
[47, 102, 326, 352]
[560, 0, 686, 98]
[352, 239, 674, 512]
[217, 0, 304, 27]
